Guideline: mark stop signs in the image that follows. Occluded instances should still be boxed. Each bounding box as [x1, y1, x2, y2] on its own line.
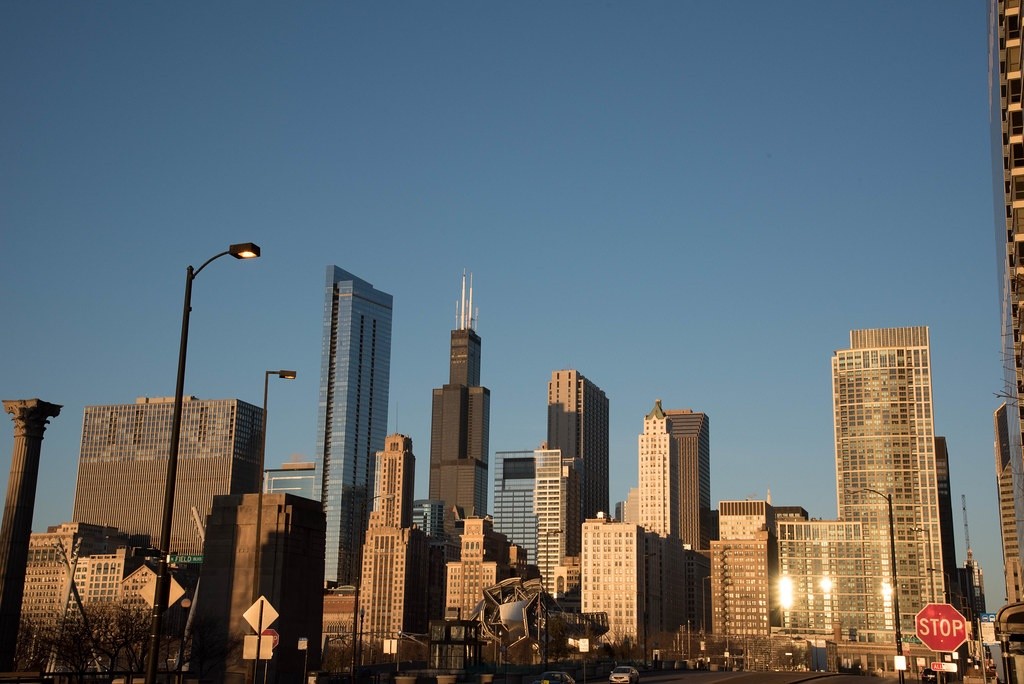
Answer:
[262, 629, 279, 650]
[915, 603, 968, 653]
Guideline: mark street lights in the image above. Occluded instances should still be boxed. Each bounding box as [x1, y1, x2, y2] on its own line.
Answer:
[349, 495, 396, 684]
[358, 606, 365, 667]
[544, 530, 563, 672]
[252, 370, 296, 602]
[143, 242, 263, 684]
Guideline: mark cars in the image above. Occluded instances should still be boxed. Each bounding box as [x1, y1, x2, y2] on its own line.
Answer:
[920, 668, 944, 684]
[536, 671, 575, 684]
[610, 666, 639, 684]
